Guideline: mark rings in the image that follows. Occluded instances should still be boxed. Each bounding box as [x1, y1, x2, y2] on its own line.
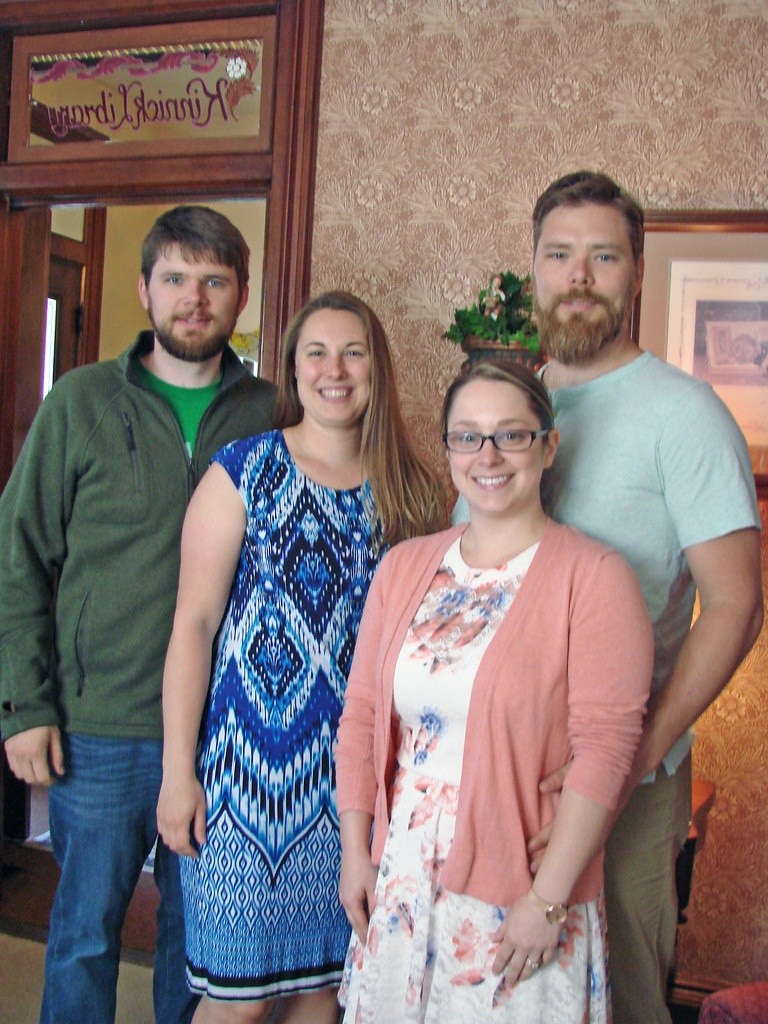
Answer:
[526, 956, 542, 968]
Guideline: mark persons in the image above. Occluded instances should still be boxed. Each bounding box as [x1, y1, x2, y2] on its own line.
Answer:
[451, 171, 765, 1023]
[0, 206, 280, 1024]
[335, 360, 655, 1024]
[156, 292, 442, 1024]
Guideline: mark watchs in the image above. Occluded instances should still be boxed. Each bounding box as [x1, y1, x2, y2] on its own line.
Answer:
[527, 888, 569, 925]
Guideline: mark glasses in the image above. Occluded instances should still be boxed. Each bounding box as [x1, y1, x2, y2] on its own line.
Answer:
[442, 430, 548, 452]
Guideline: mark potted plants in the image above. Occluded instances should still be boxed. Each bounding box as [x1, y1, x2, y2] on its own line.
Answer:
[445, 273, 548, 384]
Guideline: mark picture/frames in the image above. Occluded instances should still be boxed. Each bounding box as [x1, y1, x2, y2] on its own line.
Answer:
[630, 211, 766, 502]
